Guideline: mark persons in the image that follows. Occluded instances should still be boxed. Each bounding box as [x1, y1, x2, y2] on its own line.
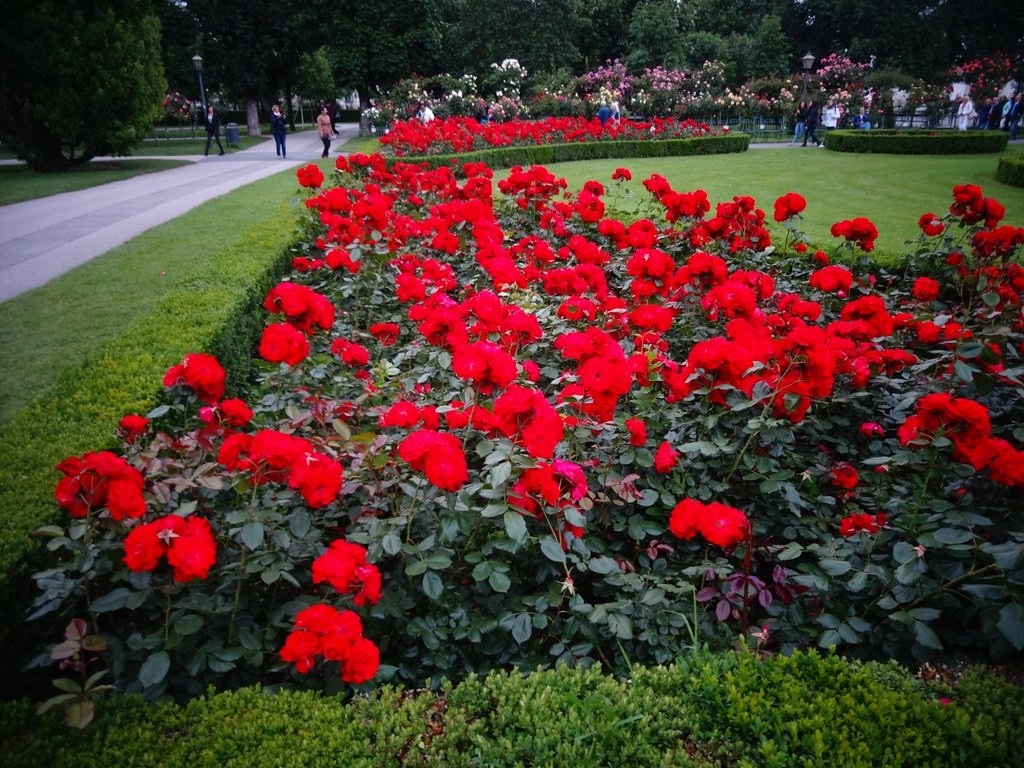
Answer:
[407, 102, 436, 127]
[977, 92, 1024, 141]
[317, 99, 338, 138]
[854, 108, 870, 130]
[800, 100, 825, 147]
[596, 100, 620, 127]
[789, 101, 809, 146]
[820, 99, 840, 147]
[318, 107, 335, 157]
[479, 107, 496, 125]
[272, 105, 287, 159]
[957, 95, 973, 132]
[205, 105, 225, 157]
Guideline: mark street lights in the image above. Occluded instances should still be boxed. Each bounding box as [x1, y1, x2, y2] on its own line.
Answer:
[801, 49, 815, 103]
[193, 50, 208, 118]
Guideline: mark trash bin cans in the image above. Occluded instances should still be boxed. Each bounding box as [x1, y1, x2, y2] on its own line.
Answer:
[224, 122, 240, 147]
